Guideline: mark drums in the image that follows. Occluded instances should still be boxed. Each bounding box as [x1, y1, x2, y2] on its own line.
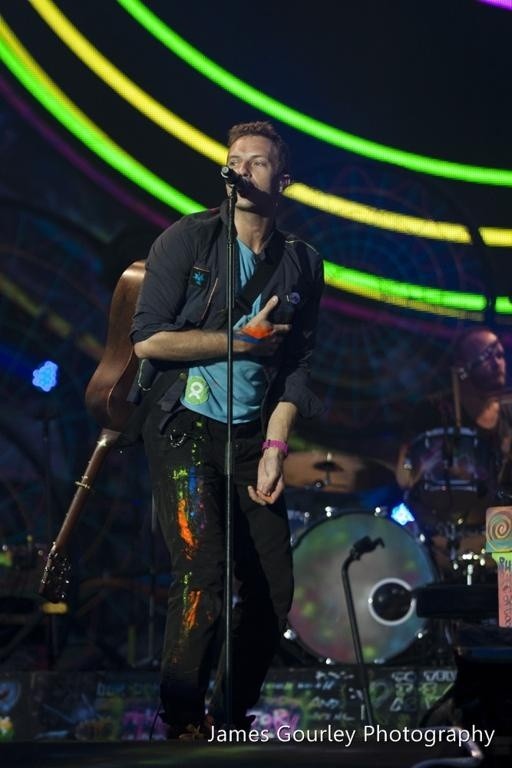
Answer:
[396, 427, 502, 514]
[290, 512, 440, 664]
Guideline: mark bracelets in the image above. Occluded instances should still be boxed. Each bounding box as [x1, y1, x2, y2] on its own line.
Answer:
[263, 439, 288, 451]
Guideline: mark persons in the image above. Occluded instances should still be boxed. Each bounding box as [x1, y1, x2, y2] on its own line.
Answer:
[397, 329, 512, 572]
[133, 128, 322, 741]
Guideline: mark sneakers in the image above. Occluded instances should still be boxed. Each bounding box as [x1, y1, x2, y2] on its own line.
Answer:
[160, 710, 256, 740]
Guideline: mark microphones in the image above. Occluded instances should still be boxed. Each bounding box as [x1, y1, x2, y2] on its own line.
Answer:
[220, 166, 254, 198]
[456, 340, 500, 382]
[368, 577, 418, 627]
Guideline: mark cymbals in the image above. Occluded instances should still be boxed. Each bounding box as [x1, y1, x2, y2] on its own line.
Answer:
[282, 451, 369, 495]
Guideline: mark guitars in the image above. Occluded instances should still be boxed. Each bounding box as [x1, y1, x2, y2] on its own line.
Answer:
[39, 259, 149, 602]
